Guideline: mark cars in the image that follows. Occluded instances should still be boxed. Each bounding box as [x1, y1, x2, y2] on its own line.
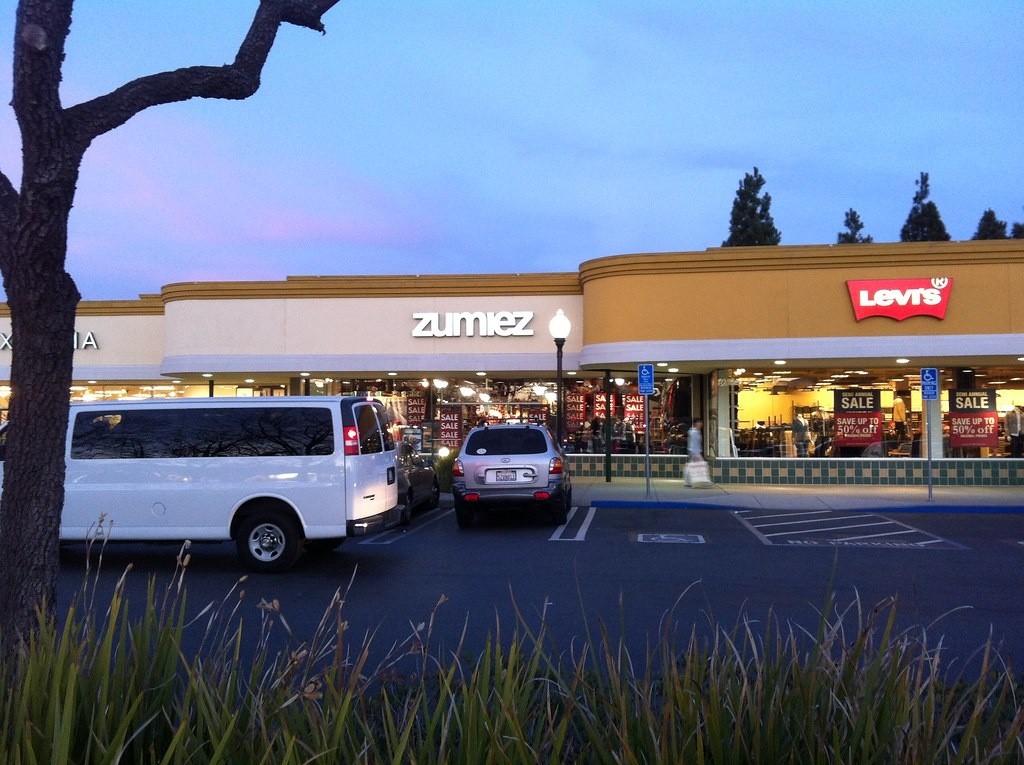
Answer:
[395, 441, 441, 529]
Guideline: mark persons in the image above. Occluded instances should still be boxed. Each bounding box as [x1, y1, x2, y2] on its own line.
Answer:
[792, 413, 810, 457]
[893, 395, 908, 442]
[1005, 407, 1024, 457]
[683, 418, 714, 488]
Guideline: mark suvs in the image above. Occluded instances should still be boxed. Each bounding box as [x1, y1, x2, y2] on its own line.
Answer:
[452, 419, 575, 529]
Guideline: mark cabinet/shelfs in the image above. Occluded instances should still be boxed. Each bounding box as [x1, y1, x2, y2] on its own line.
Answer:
[341, 378, 665, 456]
[880, 411, 949, 433]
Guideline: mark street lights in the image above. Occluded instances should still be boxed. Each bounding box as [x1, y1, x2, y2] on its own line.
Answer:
[548, 308, 572, 447]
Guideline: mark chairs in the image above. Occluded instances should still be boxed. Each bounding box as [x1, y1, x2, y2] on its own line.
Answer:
[734, 411, 834, 459]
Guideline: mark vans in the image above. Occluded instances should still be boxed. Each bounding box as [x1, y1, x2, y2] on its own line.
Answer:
[0, 397, 407, 576]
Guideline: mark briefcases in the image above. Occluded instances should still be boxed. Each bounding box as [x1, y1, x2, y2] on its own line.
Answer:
[683, 461, 713, 487]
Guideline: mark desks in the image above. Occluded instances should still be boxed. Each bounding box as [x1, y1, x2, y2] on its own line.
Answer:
[888, 449, 910, 456]
